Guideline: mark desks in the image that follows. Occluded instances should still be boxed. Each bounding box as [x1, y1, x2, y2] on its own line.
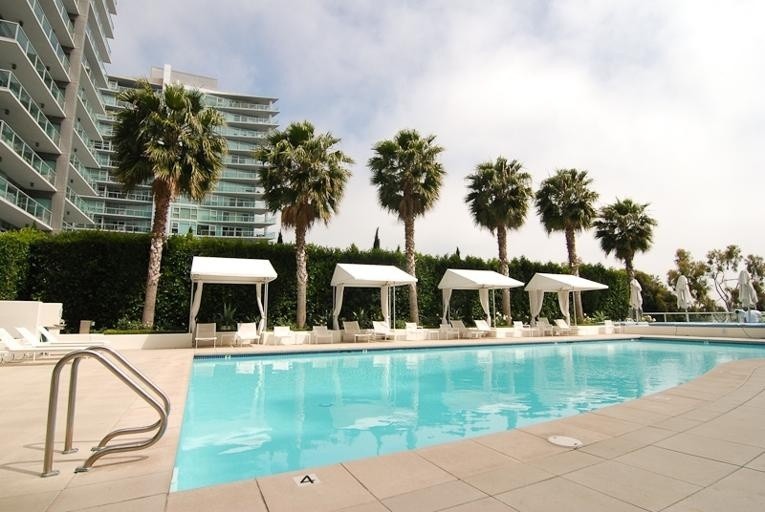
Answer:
[293, 331, 312, 344]
[221, 335, 239, 347]
[425, 329, 440, 340]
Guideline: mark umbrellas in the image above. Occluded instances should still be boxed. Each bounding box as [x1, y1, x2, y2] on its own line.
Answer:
[629, 279, 643, 325]
[676, 276, 693, 322]
[738, 270, 758, 323]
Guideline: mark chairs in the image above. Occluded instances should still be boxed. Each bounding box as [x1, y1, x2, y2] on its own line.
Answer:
[236, 322, 261, 348]
[273, 325, 293, 345]
[536, 319, 554, 338]
[526, 328, 538, 337]
[513, 320, 531, 337]
[402, 321, 425, 341]
[0, 326, 109, 362]
[311, 325, 333, 344]
[439, 323, 460, 339]
[372, 321, 400, 342]
[475, 319, 507, 338]
[554, 320, 574, 337]
[450, 320, 487, 340]
[194, 323, 218, 349]
[342, 321, 372, 344]
